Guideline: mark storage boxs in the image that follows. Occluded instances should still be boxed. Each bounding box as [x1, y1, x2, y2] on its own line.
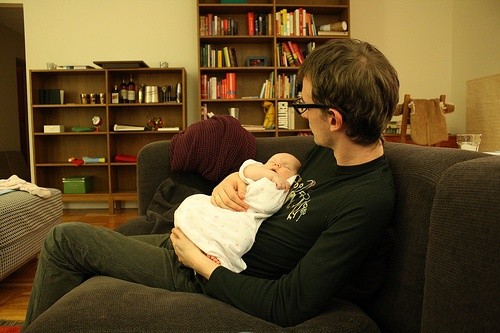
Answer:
[62, 175, 93, 194]
[43, 125, 65, 133]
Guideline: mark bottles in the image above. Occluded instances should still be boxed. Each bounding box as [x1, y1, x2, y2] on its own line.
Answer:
[80, 93, 104, 104]
[138, 83, 145, 103]
[111, 85, 120, 104]
[120, 74, 128, 103]
[128, 74, 136, 103]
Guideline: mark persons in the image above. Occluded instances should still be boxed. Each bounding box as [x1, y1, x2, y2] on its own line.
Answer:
[20, 38, 400, 333]
[171, 152, 302, 273]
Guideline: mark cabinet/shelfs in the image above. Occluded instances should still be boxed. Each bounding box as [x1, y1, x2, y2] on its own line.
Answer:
[29, 67, 187, 230]
[196, 0, 351, 135]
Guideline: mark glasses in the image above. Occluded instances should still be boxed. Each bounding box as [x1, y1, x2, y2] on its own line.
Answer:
[291, 98, 347, 122]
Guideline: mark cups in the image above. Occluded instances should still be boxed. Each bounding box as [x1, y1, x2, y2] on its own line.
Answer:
[47, 62, 55, 70]
[456, 133, 482, 151]
[160, 62, 168, 68]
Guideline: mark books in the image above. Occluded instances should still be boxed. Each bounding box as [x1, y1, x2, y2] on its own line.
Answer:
[276, 8, 349, 35]
[200, 12, 238, 36]
[200, 44, 236, 67]
[277, 41, 316, 67]
[248, 12, 273, 36]
[201, 103, 214, 120]
[276, 73, 296, 99]
[200, 73, 237, 99]
[228, 108, 240, 121]
[260, 72, 274, 98]
[278, 101, 295, 130]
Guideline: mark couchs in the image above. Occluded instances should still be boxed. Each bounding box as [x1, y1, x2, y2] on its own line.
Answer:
[24, 136, 500, 333]
[0, 185, 64, 290]
